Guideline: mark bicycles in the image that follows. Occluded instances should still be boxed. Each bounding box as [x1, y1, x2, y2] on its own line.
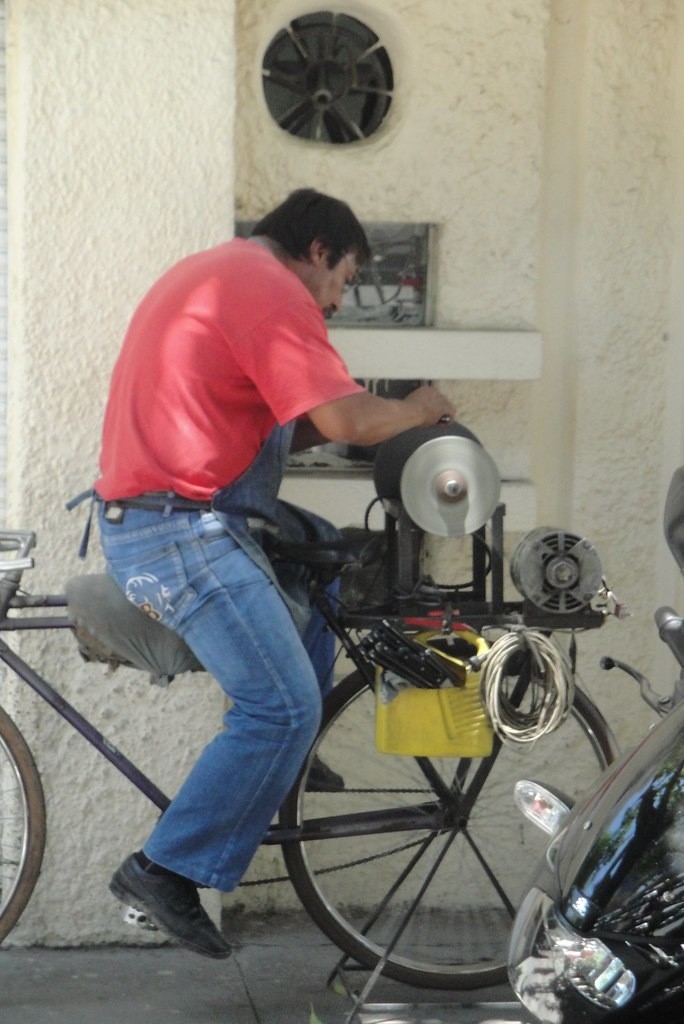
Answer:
[0, 529, 622, 1023]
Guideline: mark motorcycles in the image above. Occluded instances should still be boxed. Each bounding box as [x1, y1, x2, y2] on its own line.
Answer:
[505, 465, 683, 1024]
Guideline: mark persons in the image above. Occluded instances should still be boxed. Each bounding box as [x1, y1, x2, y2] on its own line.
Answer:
[68, 184, 462, 957]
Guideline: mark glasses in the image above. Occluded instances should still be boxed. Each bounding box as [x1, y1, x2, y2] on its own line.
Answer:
[341, 248, 362, 293]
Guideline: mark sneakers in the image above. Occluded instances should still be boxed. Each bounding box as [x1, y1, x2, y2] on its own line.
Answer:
[307, 754, 344, 789]
[110, 852, 231, 960]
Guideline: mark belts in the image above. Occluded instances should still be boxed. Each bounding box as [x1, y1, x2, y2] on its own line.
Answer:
[106, 491, 211, 512]
